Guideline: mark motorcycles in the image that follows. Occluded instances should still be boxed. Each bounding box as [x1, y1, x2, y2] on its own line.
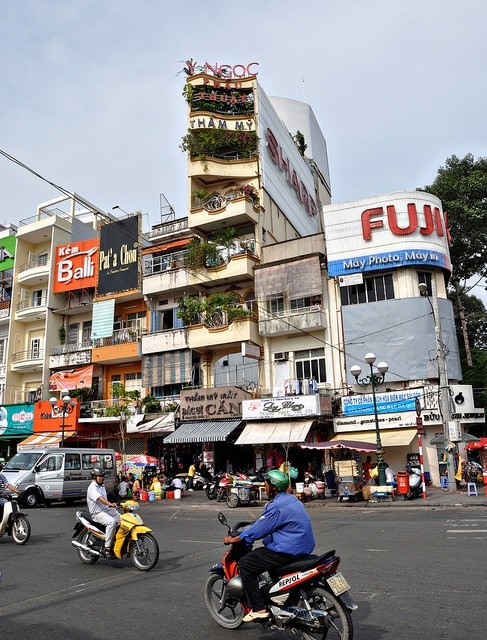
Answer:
[217, 473, 261, 501]
[185, 476, 207, 489]
[205, 512, 358, 640]
[0, 491, 31, 545]
[203, 472, 223, 500]
[72, 500, 160, 570]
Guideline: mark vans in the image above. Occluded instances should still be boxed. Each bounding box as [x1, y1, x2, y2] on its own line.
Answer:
[0, 448, 116, 508]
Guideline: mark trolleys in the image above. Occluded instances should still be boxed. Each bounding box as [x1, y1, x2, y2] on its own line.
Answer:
[338, 477, 359, 503]
[226, 484, 257, 508]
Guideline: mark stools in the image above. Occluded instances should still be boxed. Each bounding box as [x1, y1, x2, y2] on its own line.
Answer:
[441, 475, 449, 490]
[467, 482, 479, 496]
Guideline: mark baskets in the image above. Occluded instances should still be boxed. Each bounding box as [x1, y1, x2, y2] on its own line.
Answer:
[361, 483, 378, 499]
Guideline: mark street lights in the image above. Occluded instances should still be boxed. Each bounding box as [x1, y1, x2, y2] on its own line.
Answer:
[351, 353, 389, 486]
[49, 387, 76, 449]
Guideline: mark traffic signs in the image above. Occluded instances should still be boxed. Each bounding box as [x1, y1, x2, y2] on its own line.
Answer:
[415, 397, 421, 416]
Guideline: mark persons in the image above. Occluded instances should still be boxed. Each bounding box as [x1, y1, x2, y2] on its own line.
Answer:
[187, 461, 197, 492]
[127, 472, 133, 481]
[117, 476, 129, 498]
[222, 469, 315, 622]
[87, 466, 121, 561]
[385, 463, 398, 485]
[0, 463, 23, 521]
[198, 461, 205, 474]
[150, 477, 161, 492]
[130, 476, 136, 485]
[226, 460, 233, 473]
[132, 476, 144, 495]
[170, 475, 183, 490]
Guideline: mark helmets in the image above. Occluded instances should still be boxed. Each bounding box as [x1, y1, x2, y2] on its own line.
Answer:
[91, 467, 105, 477]
[264, 470, 289, 493]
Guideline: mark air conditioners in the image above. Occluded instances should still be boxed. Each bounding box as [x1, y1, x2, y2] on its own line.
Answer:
[274, 352, 288, 361]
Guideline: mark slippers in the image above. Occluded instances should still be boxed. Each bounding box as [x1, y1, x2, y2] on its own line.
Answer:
[242, 608, 270, 623]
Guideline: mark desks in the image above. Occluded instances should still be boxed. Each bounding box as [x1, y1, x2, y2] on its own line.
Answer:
[175, 472, 188, 483]
